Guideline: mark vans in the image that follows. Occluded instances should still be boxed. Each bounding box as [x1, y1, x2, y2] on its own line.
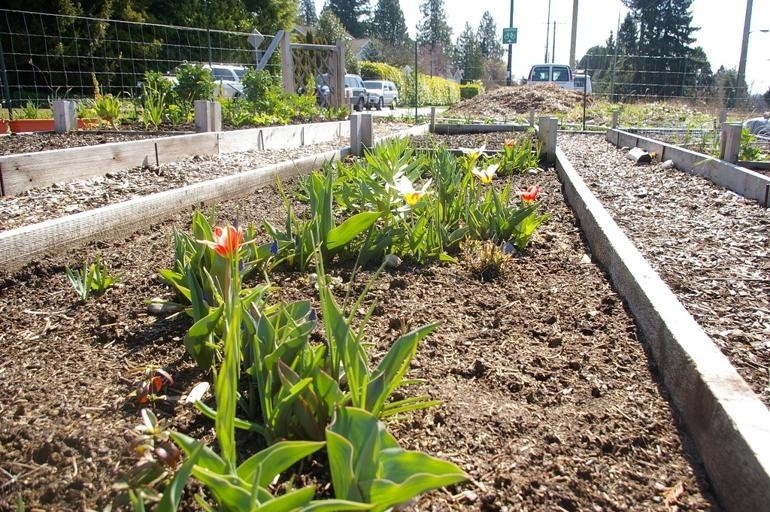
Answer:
[572, 73, 594, 96]
[529, 64, 573, 90]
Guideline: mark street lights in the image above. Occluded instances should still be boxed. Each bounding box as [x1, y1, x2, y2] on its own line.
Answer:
[733, 28, 768, 109]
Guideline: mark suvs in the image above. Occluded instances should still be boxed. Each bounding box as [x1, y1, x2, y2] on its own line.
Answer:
[363, 80, 400, 110]
[135, 61, 244, 101]
[299, 72, 368, 111]
